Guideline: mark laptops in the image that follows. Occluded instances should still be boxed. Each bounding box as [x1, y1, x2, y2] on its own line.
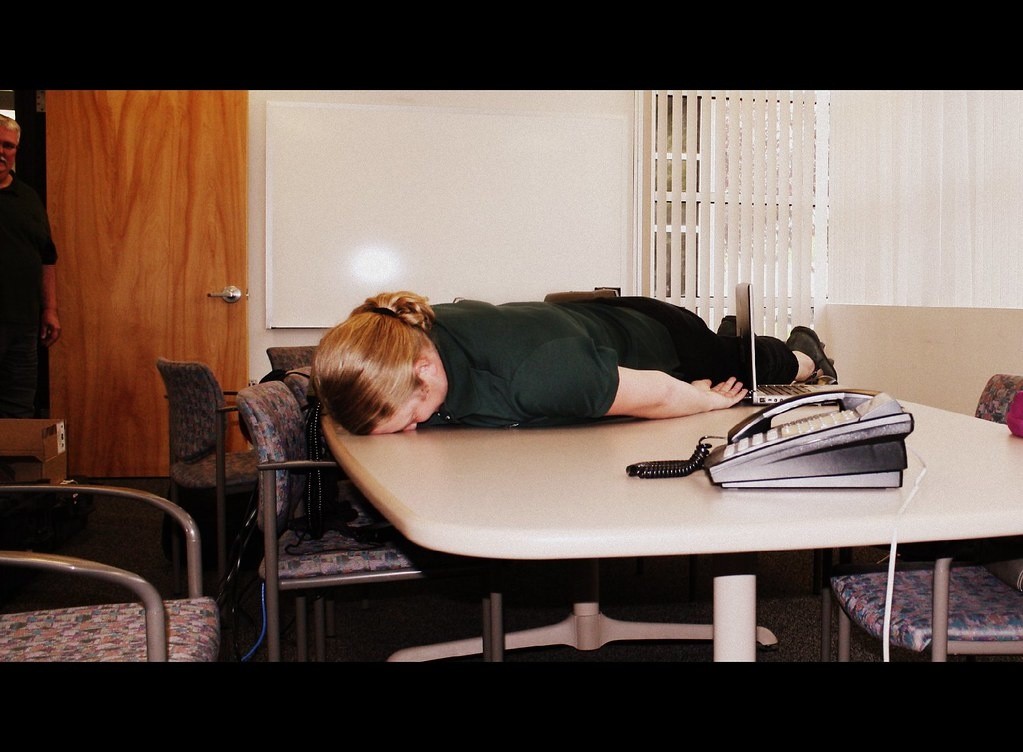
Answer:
[734, 283, 839, 407]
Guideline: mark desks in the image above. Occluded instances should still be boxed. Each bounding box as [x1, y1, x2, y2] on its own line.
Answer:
[319, 374, 1023, 662]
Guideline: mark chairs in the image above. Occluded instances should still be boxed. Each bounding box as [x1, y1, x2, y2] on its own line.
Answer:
[280, 363, 399, 533]
[238, 378, 422, 663]
[0, 479, 219, 664]
[266, 345, 322, 378]
[155, 354, 263, 604]
[830, 373, 1023, 660]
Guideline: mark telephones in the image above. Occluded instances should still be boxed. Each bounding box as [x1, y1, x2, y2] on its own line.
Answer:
[703, 387, 915, 488]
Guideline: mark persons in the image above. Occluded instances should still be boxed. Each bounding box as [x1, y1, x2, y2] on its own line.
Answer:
[0, 114, 63, 419]
[306, 286, 838, 442]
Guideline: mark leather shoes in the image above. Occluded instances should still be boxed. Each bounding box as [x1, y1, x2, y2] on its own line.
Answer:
[716, 314, 759, 338]
[785, 325, 839, 387]
